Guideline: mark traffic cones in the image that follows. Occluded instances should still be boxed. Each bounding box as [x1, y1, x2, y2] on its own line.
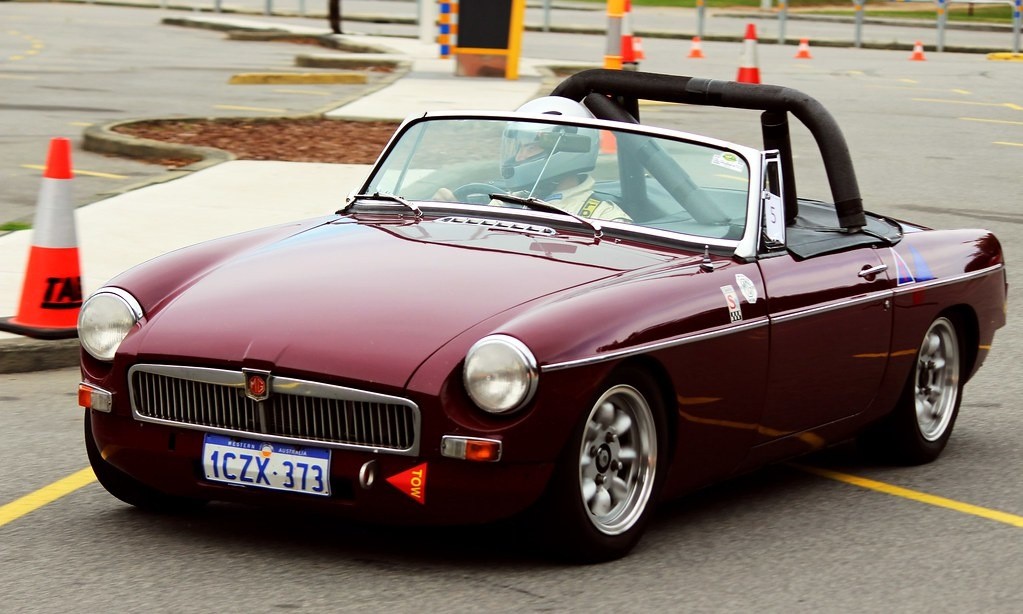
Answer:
[619, 3, 644, 66]
[737, 23, 761, 85]
[688, 35, 704, 58]
[1, 136, 84, 340]
[795, 40, 813, 59]
[910, 40, 927, 61]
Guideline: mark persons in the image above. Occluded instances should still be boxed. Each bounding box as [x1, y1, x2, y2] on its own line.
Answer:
[432, 96, 634, 224]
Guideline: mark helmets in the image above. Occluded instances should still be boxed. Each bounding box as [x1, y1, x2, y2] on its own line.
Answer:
[501, 95, 599, 187]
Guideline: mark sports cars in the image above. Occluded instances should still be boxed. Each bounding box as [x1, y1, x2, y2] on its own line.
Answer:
[78, 65, 1008, 562]
